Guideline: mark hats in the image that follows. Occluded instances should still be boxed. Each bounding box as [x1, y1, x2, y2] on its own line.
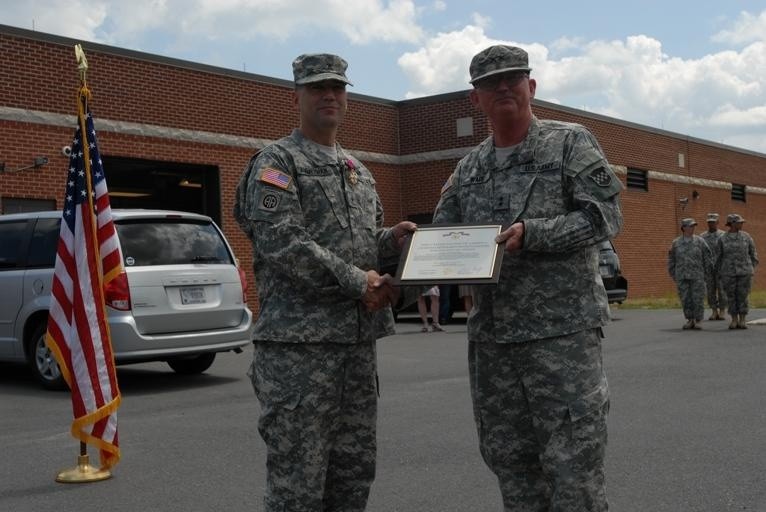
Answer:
[682, 213, 747, 226]
[292, 54, 354, 91]
[470, 45, 532, 83]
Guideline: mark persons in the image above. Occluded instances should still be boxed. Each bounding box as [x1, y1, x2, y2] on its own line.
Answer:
[440, 285, 451, 324]
[417, 284, 446, 333]
[700, 213, 728, 321]
[714, 214, 759, 329]
[459, 284, 476, 316]
[233, 53, 419, 512]
[667, 218, 713, 329]
[373, 45, 625, 512]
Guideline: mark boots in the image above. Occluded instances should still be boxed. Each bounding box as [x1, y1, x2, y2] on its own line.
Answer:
[683, 308, 748, 329]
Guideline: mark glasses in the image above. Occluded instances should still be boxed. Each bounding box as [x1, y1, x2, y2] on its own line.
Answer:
[475, 72, 530, 91]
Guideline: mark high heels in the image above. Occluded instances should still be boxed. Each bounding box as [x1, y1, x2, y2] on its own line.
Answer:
[432, 322, 445, 331]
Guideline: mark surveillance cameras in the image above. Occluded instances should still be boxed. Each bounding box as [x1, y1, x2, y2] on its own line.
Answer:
[63, 147, 73, 155]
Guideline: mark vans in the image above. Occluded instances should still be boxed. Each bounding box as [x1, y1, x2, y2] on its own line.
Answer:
[0, 203, 258, 399]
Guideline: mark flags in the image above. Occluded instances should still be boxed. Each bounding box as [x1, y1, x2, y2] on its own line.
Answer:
[45, 83, 133, 471]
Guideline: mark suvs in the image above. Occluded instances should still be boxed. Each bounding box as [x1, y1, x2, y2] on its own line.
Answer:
[594, 237, 630, 307]
